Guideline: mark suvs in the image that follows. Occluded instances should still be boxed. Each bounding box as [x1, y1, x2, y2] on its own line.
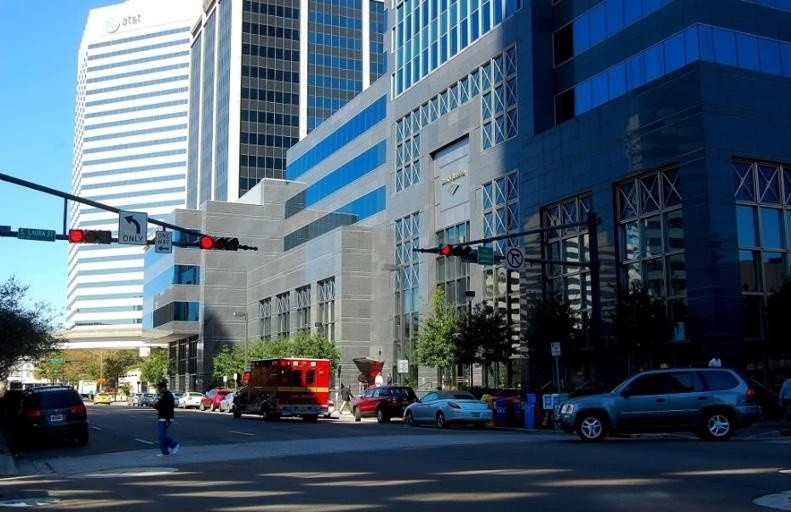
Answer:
[198, 387, 233, 413]
[552, 365, 764, 444]
[351, 386, 420, 425]
[1, 382, 91, 448]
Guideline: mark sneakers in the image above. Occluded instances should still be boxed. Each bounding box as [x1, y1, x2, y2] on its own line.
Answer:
[172, 444, 179, 455]
[157, 453, 168, 457]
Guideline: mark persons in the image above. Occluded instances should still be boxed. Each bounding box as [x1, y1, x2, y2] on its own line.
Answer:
[664, 361, 672, 368]
[404, 381, 410, 387]
[435, 387, 441, 390]
[385, 372, 394, 386]
[778, 376, 791, 425]
[374, 372, 383, 387]
[337, 384, 357, 415]
[707, 351, 723, 368]
[358, 369, 368, 395]
[574, 370, 586, 390]
[147, 383, 180, 458]
[660, 362, 665, 369]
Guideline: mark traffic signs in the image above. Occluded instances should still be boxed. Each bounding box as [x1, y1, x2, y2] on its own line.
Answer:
[17, 227, 58, 242]
[477, 245, 495, 266]
[118, 210, 147, 245]
[50, 357, 65, 362]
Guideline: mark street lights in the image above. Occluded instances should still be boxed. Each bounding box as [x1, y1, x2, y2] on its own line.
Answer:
[465, 288, 477, 385]
[68, 228, 113, 245]
[154, 230, 173, 255]
[199, 235, 241, 252]
[233, 311, 249, 369]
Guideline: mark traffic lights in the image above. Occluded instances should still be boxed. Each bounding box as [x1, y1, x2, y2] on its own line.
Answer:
[438, 242, 473, 259]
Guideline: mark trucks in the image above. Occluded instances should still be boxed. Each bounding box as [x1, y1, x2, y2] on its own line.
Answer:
[228, 354, 333, 423]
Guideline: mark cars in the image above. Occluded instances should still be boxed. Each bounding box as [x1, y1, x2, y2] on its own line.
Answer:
[127, 389, 207, 409]
[217, 391, 234, 413]
[93, 392, 112, 406]
[402, 389, 494, 429]
